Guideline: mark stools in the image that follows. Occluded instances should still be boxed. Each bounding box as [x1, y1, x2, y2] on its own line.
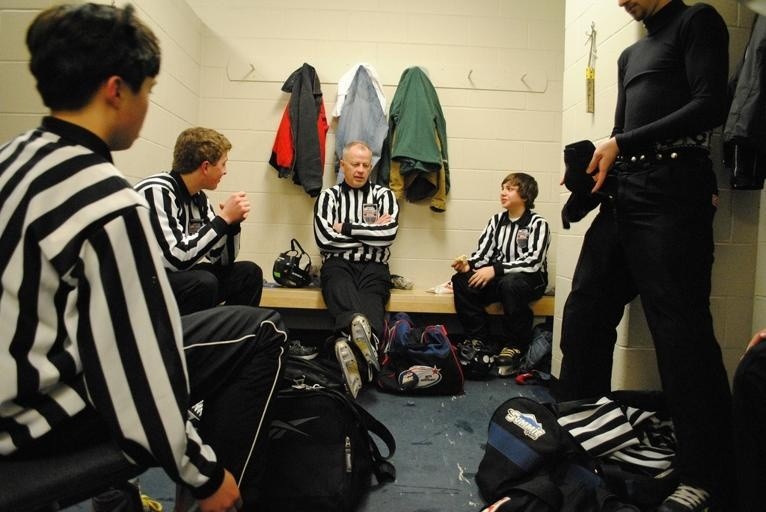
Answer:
[0, 435, 196, 512]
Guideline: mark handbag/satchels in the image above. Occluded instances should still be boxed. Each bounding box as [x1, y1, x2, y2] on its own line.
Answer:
[476, 397, 674, 512]
[252, 341, 372, 511]
[376, 313, 464, 396]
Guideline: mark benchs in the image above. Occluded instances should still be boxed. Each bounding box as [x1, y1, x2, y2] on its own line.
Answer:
[260, 284, 554, 317]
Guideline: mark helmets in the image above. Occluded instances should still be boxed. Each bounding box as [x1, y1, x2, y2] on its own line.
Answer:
[272, 254, 312, 288]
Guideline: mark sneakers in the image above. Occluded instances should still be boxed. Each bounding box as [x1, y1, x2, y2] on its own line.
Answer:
[658, 482, 714, 512]
[285, 338, 320, 360]
[92, 477, 163, 512]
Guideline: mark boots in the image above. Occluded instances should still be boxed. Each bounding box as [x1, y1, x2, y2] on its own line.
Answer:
[336, 312, 380, 399]
[497, 346, 521, 368]
[457, 339, 486, 365]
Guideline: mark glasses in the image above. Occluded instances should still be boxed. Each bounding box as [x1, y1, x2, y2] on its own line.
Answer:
[351, 162, 370, 169]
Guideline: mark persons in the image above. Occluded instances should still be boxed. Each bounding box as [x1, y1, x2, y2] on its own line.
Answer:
[554, 2, 737, 511]
[132, 125, 263, 317]
[449, 172, 554, 379]
[311, 139, 402, 404]
[1, 0, 290, 510]
